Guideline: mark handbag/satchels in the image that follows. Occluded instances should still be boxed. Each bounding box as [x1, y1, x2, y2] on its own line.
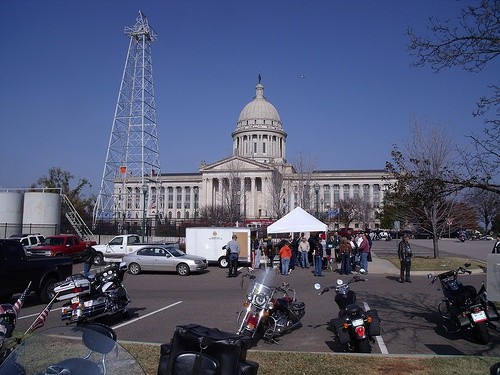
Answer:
[157, 324, 259, 375]
[288, 302, 306, 321]
[328, 318, 351, 344]
[367, 309, 381, 335]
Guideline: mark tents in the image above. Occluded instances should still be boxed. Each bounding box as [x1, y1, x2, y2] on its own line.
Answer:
[266, 205, 328, 238]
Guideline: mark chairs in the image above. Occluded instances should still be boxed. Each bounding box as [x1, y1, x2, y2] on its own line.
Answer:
[159, 250, 162, 256]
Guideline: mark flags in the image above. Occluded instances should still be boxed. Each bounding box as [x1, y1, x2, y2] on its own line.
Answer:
[29, 295, 57, 332]
[11, 285, 30, 324]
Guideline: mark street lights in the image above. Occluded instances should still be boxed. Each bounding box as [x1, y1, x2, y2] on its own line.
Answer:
[141, 181, 150, 244]
[314, 183, 320, 219]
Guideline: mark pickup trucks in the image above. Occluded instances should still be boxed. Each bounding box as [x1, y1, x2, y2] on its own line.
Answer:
[89, 233, 179, 267]
[0, 238, 74, 309]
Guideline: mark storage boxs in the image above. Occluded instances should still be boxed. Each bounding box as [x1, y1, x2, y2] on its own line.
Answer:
[481, 299, 500, 321]
[53, 278, 91, 301]
[330, 317, 352, 344]
[156, 323, 260, 375]
[365, 308, 383, 337]
[450, 303, 471, 331]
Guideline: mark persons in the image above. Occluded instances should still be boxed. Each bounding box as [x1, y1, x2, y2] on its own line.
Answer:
[226, 235, 240, 278]
[492, 235, 500, 254]
[398, 234, 413, 283]
[254, 235, 262, 269]
[263, 229, 372, 277]
[79, 242, 97, 279]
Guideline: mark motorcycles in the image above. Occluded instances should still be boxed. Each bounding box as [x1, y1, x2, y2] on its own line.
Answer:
[0, 321, 147, 375]
[51, 254, 132, 329]
[457, 229, 468, 242]
[233, 262, 306, 350]
[313, 263, 384, 353]
[426, 261, 500, 345]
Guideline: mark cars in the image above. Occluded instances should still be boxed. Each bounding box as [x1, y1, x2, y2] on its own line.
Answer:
[30, 234, 98, 264]
[122, 244, 209, 276]
[10, 233, 46, 257]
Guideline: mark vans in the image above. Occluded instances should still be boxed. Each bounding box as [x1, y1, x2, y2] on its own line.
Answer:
[333, 227, 439, 241]
